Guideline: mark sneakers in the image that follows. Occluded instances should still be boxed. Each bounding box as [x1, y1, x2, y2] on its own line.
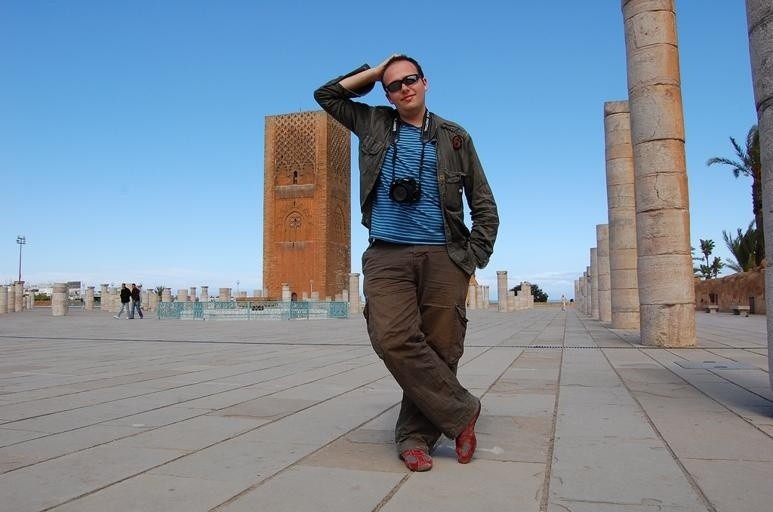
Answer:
[457, 402, 483, 464]
[400, 450, 433, 471]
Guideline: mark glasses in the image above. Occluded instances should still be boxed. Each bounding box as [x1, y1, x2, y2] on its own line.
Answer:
[386, 74, 422, 93]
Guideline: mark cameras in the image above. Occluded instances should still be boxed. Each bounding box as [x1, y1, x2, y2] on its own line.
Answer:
[389, 176, 421, 204]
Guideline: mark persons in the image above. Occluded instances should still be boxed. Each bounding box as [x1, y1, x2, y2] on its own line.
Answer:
[129, 284, 143, 319]
[113, 283, 132, 319]
[562, 294, 565, 311]
[315, 54, 500, 471]
